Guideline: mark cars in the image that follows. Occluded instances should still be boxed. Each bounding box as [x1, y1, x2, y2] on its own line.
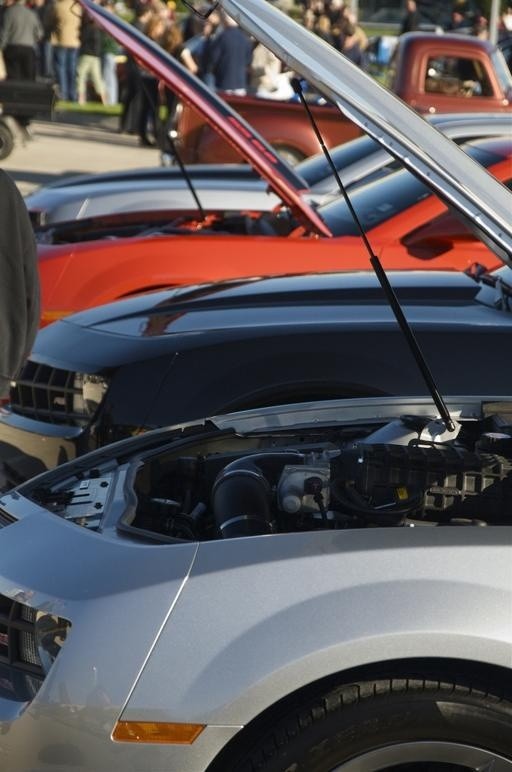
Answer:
[21, 110, 512, 230]
[0, 1, 512, 333]
[0, 258, 512, 491]
[0, 1, 512, 772]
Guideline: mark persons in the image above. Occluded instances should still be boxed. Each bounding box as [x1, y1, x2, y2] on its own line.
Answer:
[98, 0, 129, 106]
[439, 2, 512, 68]
[396, 0, 423, 35]
[0, 0, 42, 82]
[76, 7, 109, 108]
[44, 0, 84, 104]
[118, 0, 370, 156]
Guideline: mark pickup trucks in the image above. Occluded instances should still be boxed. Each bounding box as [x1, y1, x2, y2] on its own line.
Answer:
[176, 33, 512, 167]
